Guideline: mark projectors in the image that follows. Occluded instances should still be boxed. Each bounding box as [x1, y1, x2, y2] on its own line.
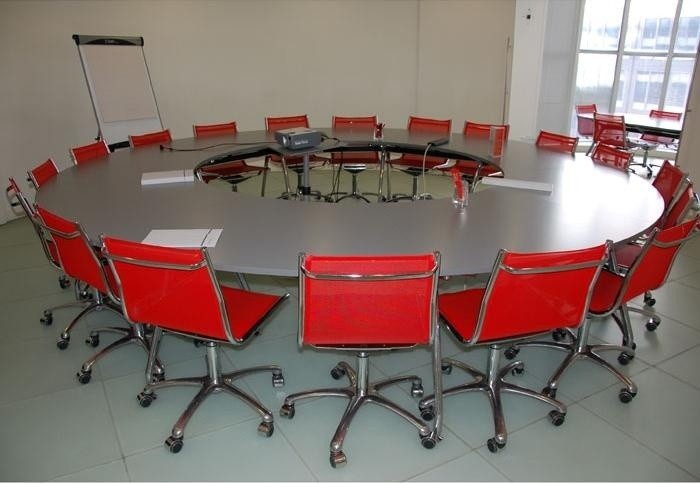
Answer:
[274, 127, 323, 150]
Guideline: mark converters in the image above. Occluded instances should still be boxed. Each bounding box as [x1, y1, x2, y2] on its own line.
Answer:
[428, 138, 448, 146]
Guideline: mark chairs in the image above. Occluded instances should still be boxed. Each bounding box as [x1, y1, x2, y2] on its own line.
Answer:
[6, 104, 700, 468]
